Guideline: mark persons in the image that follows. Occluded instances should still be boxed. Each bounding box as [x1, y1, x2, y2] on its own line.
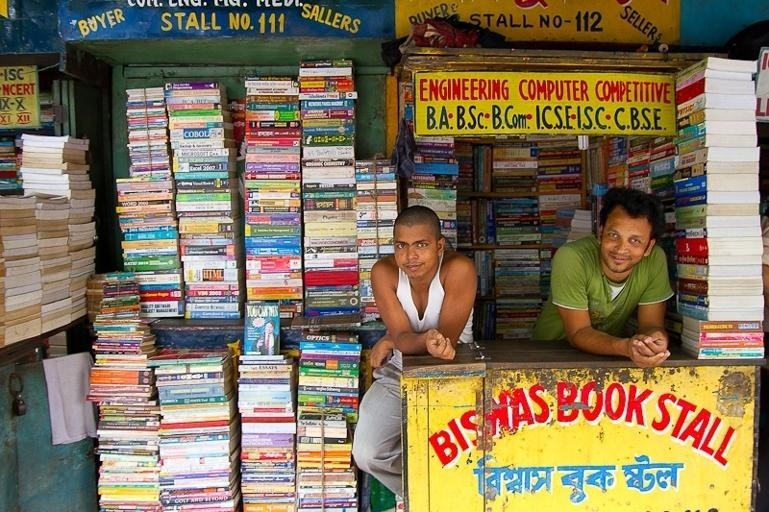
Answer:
[350, 204, 477, 512]
[255, 319, 279, 355]
[536, 189, 675, 369]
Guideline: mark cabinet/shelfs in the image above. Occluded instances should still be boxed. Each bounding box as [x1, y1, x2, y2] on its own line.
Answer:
[453, 136, 606, 301]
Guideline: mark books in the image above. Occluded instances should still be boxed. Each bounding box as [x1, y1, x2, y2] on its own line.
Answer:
[389, 57, 764, 359]
[114, 59, 399, 323]
[0, 133, 97, 358]
[85, 272, 362, 512]
